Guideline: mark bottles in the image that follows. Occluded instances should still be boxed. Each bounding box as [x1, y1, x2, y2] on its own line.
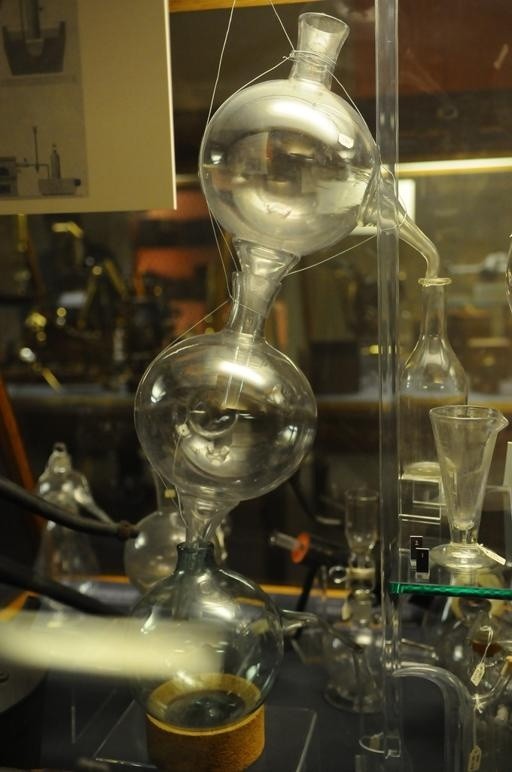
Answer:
[402, 277, 469, 476]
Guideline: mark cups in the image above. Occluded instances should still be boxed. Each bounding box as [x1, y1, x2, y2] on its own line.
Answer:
[429, 405, 509, 568]
